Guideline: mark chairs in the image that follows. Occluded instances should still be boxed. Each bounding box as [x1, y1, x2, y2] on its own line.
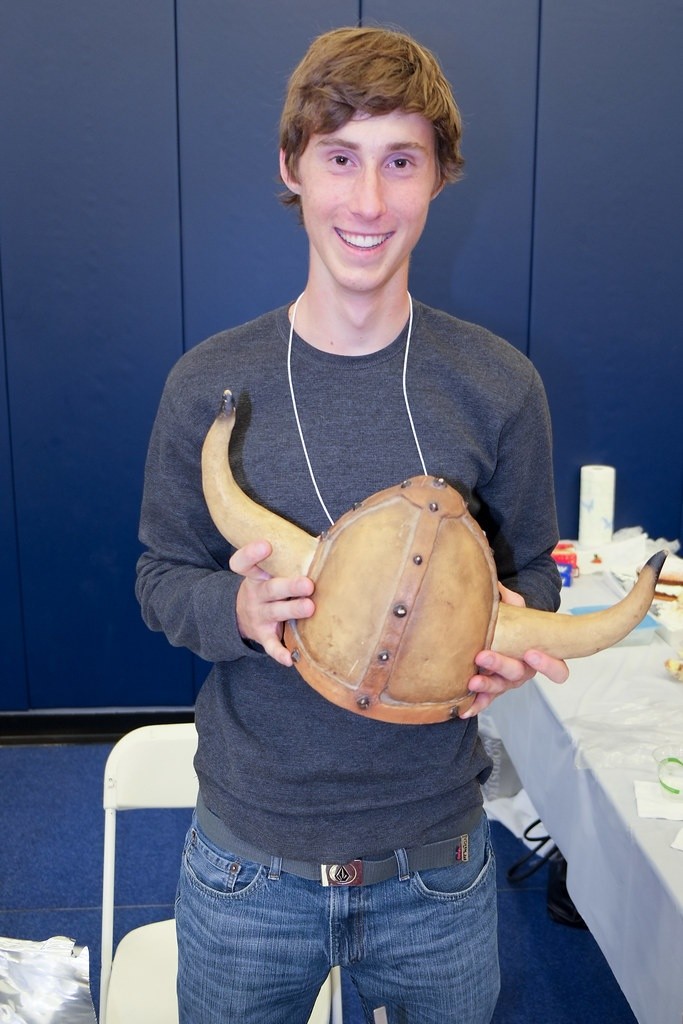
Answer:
[99, 721, 343, 1024]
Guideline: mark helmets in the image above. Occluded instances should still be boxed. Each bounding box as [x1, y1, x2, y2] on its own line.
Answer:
[199, 387, 670, 724]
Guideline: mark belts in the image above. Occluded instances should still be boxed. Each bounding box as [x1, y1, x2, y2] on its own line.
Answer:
[193, 791, 484, 887]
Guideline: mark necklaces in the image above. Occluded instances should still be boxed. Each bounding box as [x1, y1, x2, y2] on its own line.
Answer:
[287, 291, 427, 526]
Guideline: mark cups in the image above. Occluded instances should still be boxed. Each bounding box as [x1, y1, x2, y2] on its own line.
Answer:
[652, 745, 683, 801]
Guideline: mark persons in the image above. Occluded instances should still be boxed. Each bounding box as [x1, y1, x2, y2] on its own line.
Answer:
[135, 24, 569, 1024]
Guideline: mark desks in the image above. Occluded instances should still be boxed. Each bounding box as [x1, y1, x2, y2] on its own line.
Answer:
[479, 537, 683, 1024]
